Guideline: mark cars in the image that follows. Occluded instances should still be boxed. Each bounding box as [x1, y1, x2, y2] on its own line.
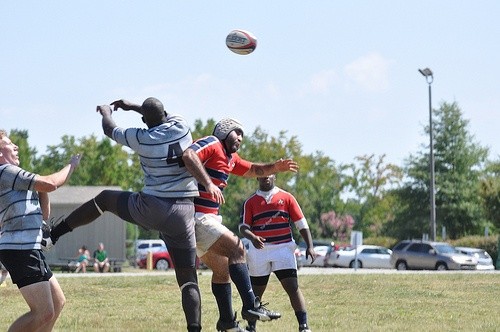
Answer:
[334, 244, 393, 268]
[296, 240, 351, 267]
[138, 250, 200, 269]
[454, 246, 495, 271]
[389, 240, 478, 271]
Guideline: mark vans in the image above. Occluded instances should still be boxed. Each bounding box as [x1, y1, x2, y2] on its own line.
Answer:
[135, 239, 166, 254]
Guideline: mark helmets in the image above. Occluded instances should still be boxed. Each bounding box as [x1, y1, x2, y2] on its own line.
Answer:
[212, 117, 244, 157]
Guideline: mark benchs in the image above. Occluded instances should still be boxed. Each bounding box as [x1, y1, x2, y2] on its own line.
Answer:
[55, 257, 126, 272]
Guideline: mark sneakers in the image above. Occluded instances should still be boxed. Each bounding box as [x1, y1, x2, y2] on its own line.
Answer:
[299, 324, 311, 332]
[40, 220, 54, 252]
[216, 319, 251, 332]
[241, 304, 282, 322]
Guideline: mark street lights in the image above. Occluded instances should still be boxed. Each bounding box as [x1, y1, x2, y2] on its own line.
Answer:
[418, 67, 436, 243]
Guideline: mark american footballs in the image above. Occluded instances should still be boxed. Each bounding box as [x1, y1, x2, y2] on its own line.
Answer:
[226, 29, 258, 56]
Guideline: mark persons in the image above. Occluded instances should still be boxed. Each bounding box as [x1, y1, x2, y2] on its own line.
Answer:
[75, 242, 110, 274]
[0, 128, 84, 332]
[40, 97, 202, 332]
[239, 173, 317, 332]
[182, 118, 300, 332]
